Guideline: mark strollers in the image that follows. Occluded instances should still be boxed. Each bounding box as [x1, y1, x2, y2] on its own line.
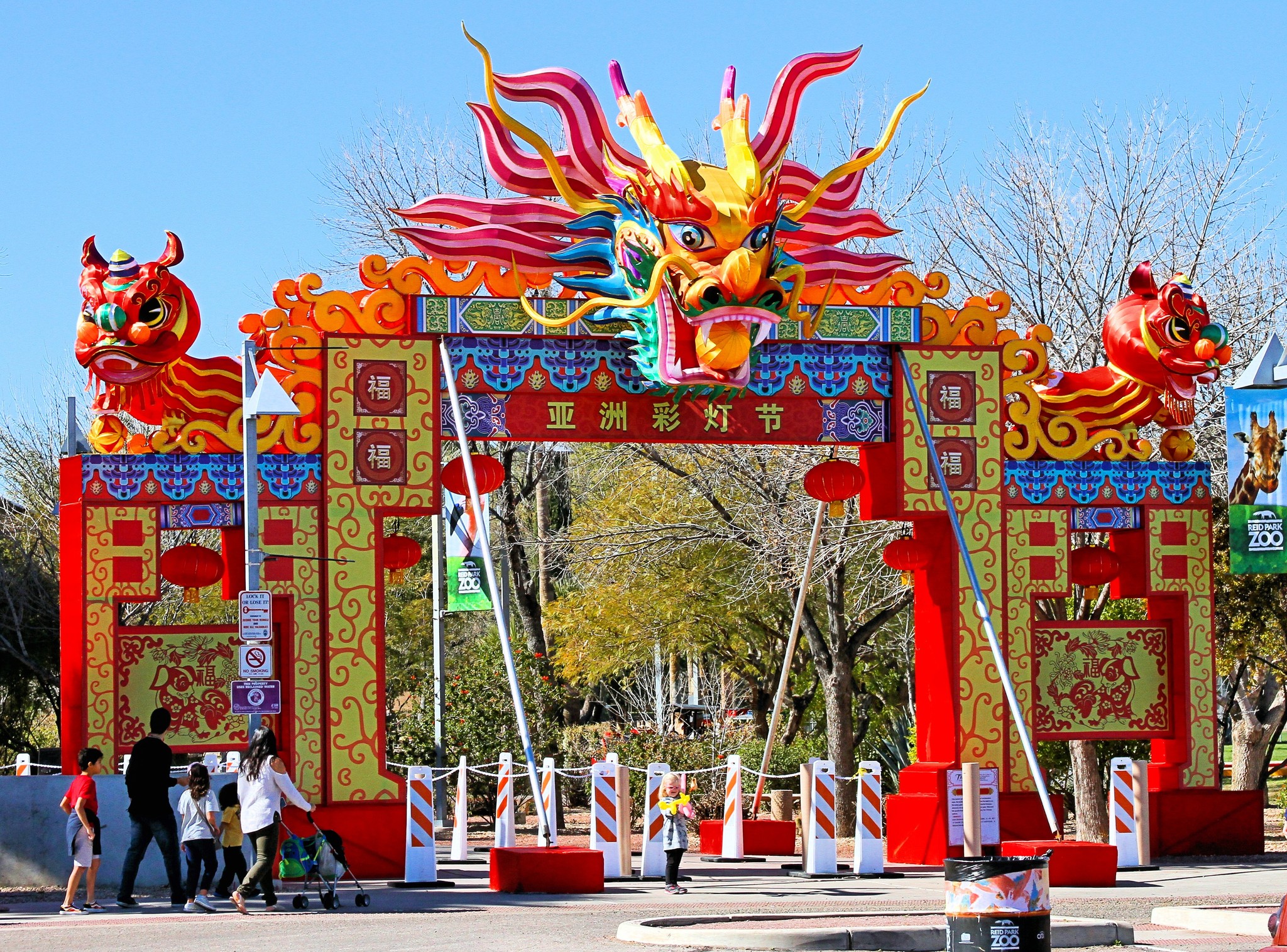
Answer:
[278, 811, 370, 910]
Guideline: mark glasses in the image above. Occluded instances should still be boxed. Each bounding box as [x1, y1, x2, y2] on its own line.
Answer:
[665, 785, 680, 790]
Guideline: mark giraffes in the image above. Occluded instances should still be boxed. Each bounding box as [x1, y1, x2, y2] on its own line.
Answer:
[1229, 409, 1287, 507]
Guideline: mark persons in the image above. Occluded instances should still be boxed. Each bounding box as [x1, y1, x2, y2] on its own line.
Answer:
[658, 772, 688, 894]
[59, 748, 107, 916]
[115, 707, 190, 907]
[177, 726, 317, 914]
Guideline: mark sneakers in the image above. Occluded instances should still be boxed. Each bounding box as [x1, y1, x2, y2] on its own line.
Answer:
[83, 900, 107, 913]
[664, 884, 679, 894]
[251, 888, 261, 897]
[673, 884, 687, 894]
[214, 886, 233, 898]
[183, 902, 205, 913]
[171, 901, 187, 907]
[194, 894, 217, 912]
[59, 902, 89, 915]
[116, 897, 139, 907]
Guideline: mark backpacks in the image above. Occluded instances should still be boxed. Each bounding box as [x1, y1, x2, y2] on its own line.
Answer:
[278, 834, 326, 880]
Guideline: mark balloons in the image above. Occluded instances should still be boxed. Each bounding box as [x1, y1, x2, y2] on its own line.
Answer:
[659, 792, 693, 818]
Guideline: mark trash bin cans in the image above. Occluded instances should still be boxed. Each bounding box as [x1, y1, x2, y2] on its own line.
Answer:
[942, 854, 1052, 952]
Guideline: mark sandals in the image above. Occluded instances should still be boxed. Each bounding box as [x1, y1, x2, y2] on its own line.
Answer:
[229, 891, 249, 914]
[266, 902, 285, 911]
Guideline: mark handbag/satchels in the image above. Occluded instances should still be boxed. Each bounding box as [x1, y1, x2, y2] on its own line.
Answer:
[213, 837, 222, 850]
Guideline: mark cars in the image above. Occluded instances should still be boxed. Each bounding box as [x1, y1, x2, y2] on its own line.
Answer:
[627, 712, 657, 737]
